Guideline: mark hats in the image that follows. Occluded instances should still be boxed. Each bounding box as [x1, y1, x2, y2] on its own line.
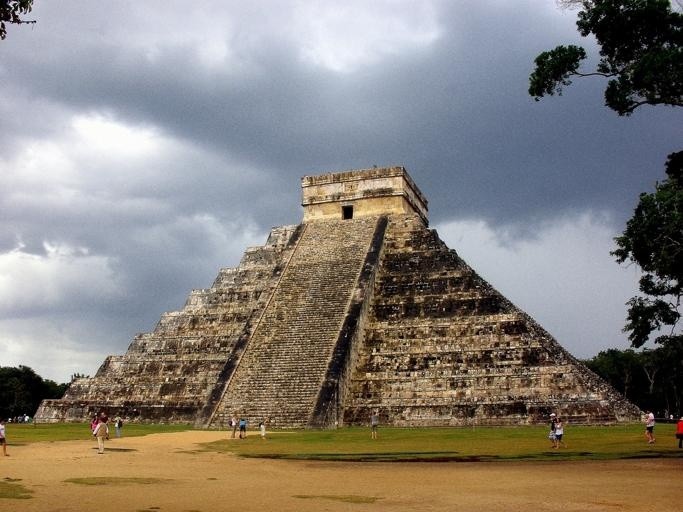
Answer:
[549, 413, 556, 417]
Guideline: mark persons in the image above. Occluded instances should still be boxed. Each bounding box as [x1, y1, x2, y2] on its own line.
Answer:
[90, 415, 99, 436]
[645, 411, 655, 444]
[0, 419, 10, 456]
[548, 412, 556, 447]
[676, 417, 682, 448]
[7, 413, 31, 423]
[553, 417, 567, 449]
[100, 412, 109, 440]
[259, 417, 267, 440]
[238, 416, 247, 439]
[111, 412, 121, 437]
[92, 417, 108, 454]
[370, 411, 377, 440]
[230, 414, 237, 438]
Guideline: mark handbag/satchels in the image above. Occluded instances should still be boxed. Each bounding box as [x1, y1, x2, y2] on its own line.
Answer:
[228, 421, 232, 427]
[118, 420, 122, 428]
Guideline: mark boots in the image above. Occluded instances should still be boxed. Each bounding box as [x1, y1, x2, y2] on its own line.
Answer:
[371, 432, 377, 440]
